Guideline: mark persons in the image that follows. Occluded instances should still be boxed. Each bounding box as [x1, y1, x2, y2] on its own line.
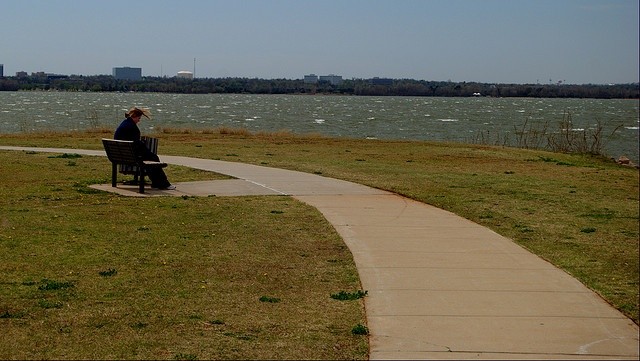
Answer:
[114, 108, 176, 190]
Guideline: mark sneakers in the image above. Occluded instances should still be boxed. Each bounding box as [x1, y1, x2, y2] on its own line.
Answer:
[159, 183, 176, 190]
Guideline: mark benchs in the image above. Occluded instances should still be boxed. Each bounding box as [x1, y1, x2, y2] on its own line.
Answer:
[101, 138, 168, 193]
